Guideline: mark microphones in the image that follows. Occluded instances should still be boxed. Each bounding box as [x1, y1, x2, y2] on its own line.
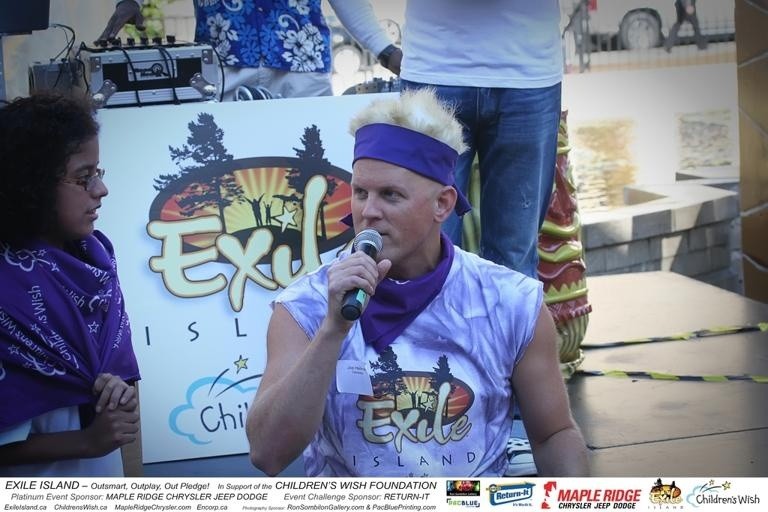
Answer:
[340, 228, 384, 321]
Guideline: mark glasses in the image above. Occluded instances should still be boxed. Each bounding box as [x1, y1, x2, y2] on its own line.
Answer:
[60, 167, 105, 191]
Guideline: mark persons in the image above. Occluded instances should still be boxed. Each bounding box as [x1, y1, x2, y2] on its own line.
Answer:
[246, 87, 589, 477]
[664, 1, 708, 51]
[0, 95, 143, 477]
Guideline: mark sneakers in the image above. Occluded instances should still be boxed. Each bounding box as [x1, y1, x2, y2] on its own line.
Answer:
[504, 437, 539, 477]
